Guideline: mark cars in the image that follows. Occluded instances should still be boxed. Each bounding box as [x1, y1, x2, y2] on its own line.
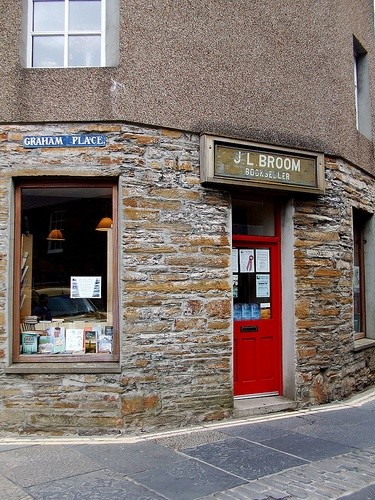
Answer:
[21, 287, 101, 321]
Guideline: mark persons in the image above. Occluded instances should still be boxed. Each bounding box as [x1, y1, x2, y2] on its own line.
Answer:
[33, 294, 52, 321]
[51, 327, 63, 343]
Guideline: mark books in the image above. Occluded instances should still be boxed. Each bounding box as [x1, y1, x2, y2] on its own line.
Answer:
[22, 327, 113, 354]
